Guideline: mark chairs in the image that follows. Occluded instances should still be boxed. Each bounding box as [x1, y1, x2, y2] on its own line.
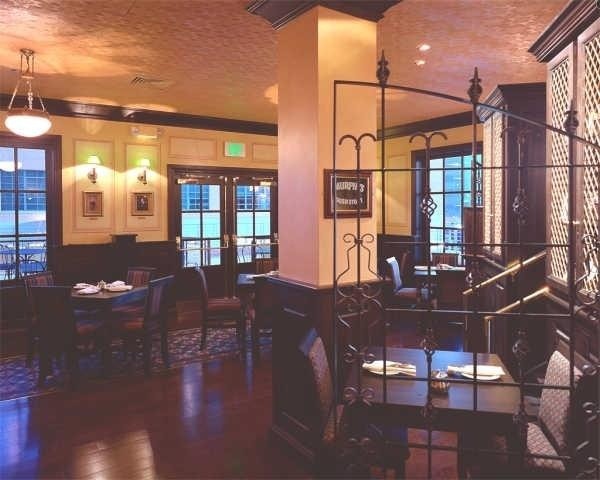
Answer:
[30, 287, 100, 384]
[119, 273, 173, 378]
[379, 252, 407, 285]
[1, 288, 34, 354]
[385, 257, 427, 323]
[431, 254, 458, 266]
[433, 270, 465, 309]
[248, 275, 277, 353]
[6, 259, 45, 280]
[466, 339, 596, 480]
[26, 271, 56, 369]
[126, 267, 158, 287]
[0, 244, 16, 280]
[21, 244, 47, 273]
[242, 244, 265, 263]
[295, 328, 410, 480]
[194, 268, 246, 358]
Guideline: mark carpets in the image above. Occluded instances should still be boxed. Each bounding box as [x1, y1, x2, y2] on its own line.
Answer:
[0, 323, 272, 404]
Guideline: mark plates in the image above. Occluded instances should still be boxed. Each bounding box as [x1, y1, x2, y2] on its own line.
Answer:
[462, 374, 501, 381]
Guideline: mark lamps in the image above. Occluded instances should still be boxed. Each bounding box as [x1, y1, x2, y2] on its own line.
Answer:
[86, 155, 102, 183]
[0, 161, 21, 172]
[137, 158, 150, 185]
[3, 47, 51, 137]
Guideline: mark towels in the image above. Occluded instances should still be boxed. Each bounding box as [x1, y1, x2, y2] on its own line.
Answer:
[447, 365, 505, 376]
[362, 359, 417, 377]
[78, 286, 101, 293]
[74, 282, 94, 288]
[107, 280, 133, 290]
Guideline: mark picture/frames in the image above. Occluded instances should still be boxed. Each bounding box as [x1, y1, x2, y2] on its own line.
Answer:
[83, 190, 104, 217]
[323, 169, 372, 217]
[130, 191, 154, 216]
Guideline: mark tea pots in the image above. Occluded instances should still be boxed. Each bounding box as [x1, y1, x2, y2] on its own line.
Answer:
[97, 280, 106, 290]
[371, 370, 398, 376]
